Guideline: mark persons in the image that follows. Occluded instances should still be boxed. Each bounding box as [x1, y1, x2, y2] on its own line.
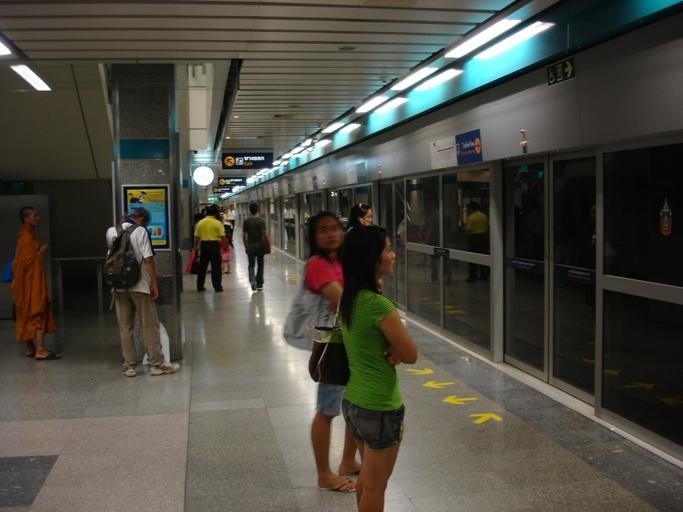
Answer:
[10, 206, 63, 360]
[425, 199, 450, 284]
[242, 202, 266, 291]
[347, 204, 383, 292]
[284, 204, 295, 241]
[334, 222, 418, 512]
[192, 203, 236, 293]
[464, 201, 488, 280]
[310, 211, 362, 494]
[104, 206, 180, 377]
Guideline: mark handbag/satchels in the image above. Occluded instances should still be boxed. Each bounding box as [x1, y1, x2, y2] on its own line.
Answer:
[185, 251, 196, 272]
[309, 326, 351, 387]
[222, 253, 232, 261]
[190, 256, 201, 274]
[283, 282, 337, 352]
[0, 246, 15, 283]
[262, 231, 271, 254]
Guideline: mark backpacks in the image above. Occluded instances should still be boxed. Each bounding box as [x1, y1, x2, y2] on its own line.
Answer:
[103, 229, 142, 289]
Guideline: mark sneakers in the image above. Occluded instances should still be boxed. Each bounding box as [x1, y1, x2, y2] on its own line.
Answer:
[150, 363, 180, 376]
[256, 281, 263, 290]
[251, 281, 257, 290]
[121, 368, 138, 378]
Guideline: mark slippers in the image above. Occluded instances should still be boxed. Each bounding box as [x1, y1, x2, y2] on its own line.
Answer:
[318, 477, 357, 493]
[35, 353, 62, 361]
[26, 350, 36, 358]
[338, 471, 362, 477]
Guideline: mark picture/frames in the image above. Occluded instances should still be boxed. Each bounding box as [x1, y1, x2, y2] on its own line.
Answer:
[121, 182, 174, 252]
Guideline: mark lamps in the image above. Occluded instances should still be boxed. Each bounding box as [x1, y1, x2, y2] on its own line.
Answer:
[192, 165, 215, 187]
